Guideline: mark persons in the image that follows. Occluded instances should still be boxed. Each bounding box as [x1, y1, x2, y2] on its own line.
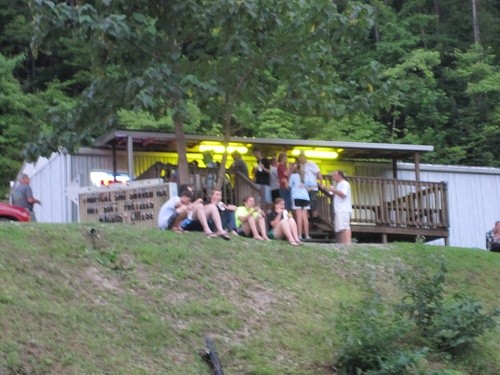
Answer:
[328, 169, 352, 245]
[11, 173, 40, 223]
[159, 150, 324, 246]
[485, 221, 500, 252]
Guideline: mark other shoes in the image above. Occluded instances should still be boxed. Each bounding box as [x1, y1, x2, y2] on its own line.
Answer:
[289, 241, 299, 246]
[206, 232, 218, 238]
[296, 240, 304, 245]
[216, 232, 231, 240]
